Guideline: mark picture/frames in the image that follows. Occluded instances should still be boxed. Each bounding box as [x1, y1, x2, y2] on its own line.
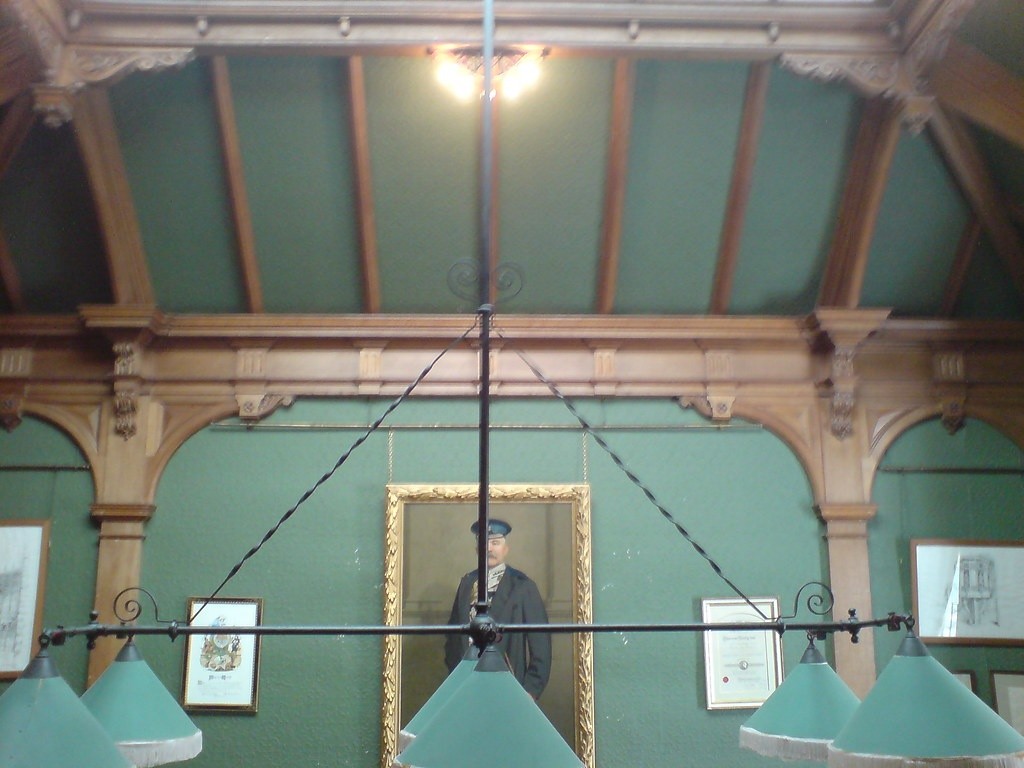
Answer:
[181, 596, 264, 713]
[0, 519, 52, 682]
[910, 538, 1024, 648]
[379, 483, 595, 768]
[700, 595, 786, 713]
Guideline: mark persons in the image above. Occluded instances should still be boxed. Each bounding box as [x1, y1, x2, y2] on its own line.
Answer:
[445, 519, 551, 702]
